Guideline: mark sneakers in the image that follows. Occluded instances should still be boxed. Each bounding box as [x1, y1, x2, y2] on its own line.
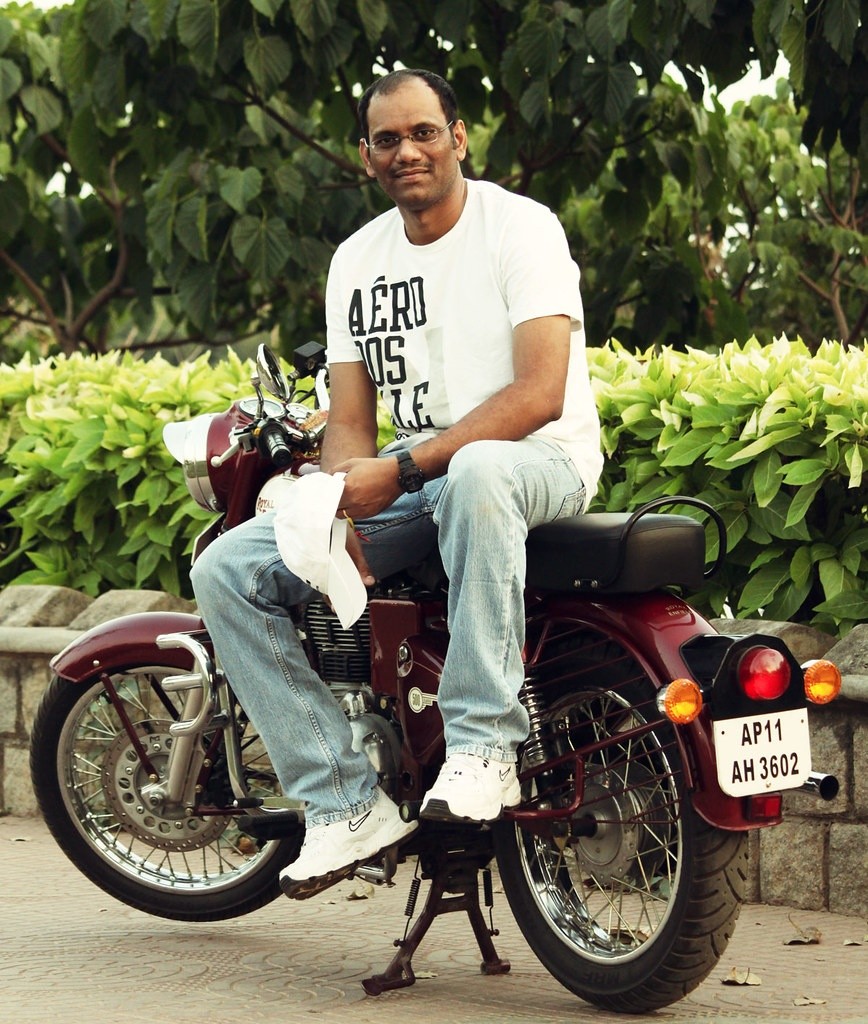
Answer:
[277, 787, 419, 899]
[421, 757, 523, 826]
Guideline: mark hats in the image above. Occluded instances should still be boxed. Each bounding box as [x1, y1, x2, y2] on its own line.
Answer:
[273, 471, 367, 631]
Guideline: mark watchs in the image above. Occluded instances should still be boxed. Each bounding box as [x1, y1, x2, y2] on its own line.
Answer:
[395, 450, 427, 493]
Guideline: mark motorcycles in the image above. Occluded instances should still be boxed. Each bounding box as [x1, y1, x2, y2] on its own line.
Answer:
[27, 344, 842, 1011]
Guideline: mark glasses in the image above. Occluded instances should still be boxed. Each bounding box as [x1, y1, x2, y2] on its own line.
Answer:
[365, 121, 454, 154]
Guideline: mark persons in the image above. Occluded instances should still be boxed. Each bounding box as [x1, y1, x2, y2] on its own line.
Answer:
[190, 71, 605, 901]
